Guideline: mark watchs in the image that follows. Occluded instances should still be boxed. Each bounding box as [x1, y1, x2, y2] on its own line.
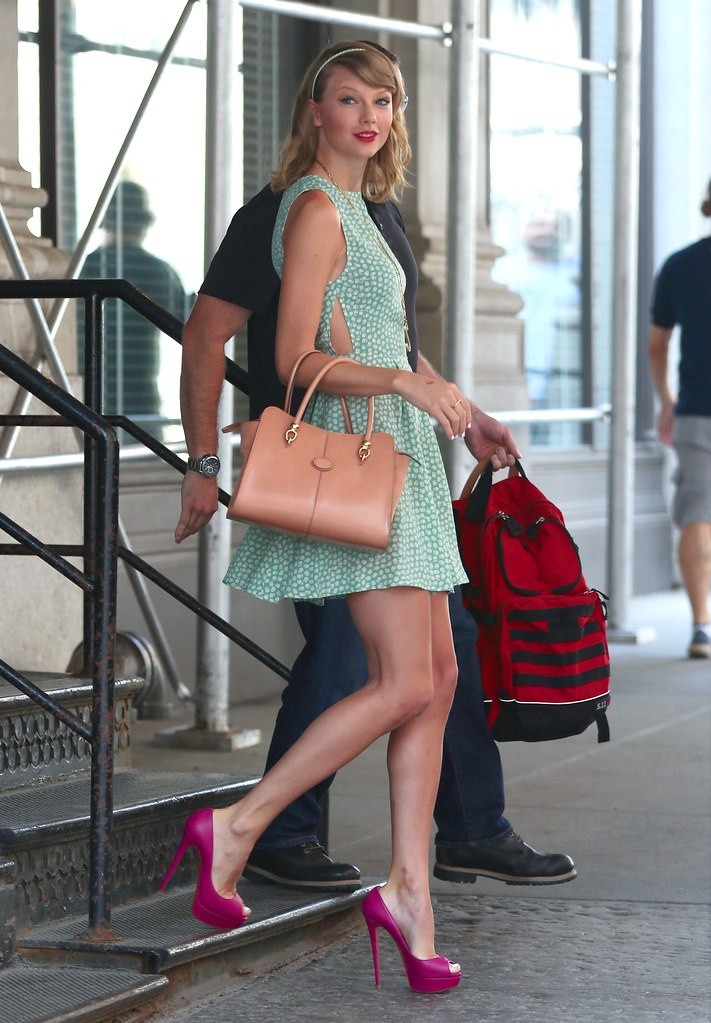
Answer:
[187, 453, 220, 478]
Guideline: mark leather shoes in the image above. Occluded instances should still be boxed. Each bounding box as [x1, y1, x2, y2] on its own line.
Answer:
[434, 826, 577, 885]
[247, 841, 362, 891]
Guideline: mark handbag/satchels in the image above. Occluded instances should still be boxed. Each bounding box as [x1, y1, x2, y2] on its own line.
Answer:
[221, 350, 410, 549]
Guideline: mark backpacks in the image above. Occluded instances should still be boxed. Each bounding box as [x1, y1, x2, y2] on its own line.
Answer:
[454, 455, 609, 743]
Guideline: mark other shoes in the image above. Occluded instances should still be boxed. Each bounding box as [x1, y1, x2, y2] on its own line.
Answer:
[689, 630, 711, 656]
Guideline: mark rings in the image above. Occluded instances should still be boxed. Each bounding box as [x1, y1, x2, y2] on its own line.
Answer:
[451, 399, 462, 408]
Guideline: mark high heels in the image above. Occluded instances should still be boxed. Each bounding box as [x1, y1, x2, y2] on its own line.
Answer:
[159, 809, 249, 929]
[361, 886, 461, 992]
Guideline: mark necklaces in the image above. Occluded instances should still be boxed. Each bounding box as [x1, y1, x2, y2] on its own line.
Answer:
[314, 158, 411, 352]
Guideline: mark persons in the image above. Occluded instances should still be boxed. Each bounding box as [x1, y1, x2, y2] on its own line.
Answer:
[77, 183, 186, 450]
[174, 182, 578, 890]
[647, 177, 711, 657]
[160, 43, 521, 992]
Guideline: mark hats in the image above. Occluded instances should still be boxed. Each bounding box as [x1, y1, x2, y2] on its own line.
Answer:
[98, 182, 155, 230]
